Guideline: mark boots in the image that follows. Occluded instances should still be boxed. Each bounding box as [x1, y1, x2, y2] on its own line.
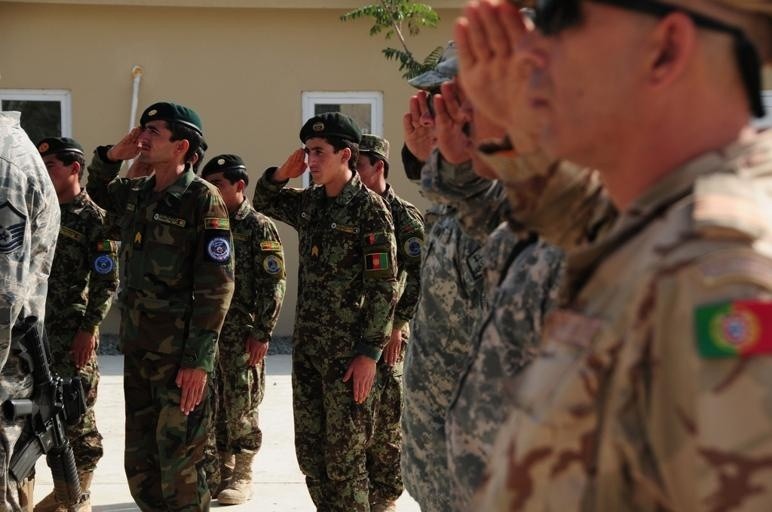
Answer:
[52, 470, 93, 512]
[211, 448, 258, 506]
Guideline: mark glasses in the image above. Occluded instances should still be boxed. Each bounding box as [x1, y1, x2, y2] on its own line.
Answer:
[534, 0, 588, 39]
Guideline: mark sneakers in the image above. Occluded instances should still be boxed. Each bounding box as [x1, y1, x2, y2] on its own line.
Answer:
[30, 485, 60, 512]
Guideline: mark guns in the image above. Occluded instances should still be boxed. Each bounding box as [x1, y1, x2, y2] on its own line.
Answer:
[4, 316, 89, 506]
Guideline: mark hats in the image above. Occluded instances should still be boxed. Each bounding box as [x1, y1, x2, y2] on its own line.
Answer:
[402, 38, 465, 90]
[139, 101, 204, 138]
[36, 136, 84, 157]
[357, 132, 391, 160]
[201, 153, 247, 179]
[298, 111, 363, 144]
